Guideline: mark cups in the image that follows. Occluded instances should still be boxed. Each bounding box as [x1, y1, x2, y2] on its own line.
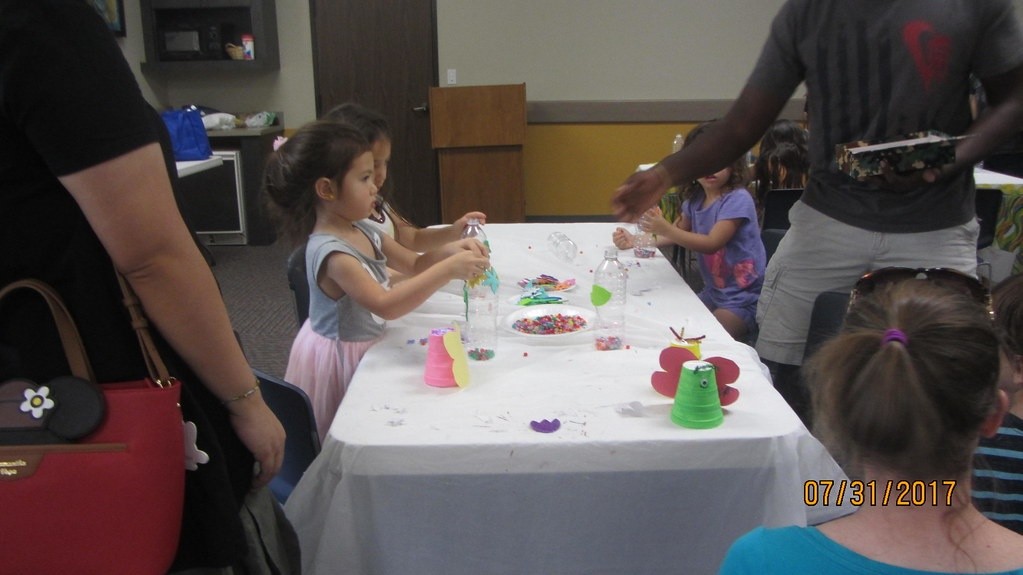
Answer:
[670, 359, 723, 429]
[669, 338, 701, 360]
[240, 35, 254, 60]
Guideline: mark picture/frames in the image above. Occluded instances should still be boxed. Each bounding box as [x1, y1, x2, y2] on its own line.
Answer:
[92, 0, 127, 39]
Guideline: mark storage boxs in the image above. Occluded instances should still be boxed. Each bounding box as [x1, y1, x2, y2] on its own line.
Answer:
[834, 127, 985, 181]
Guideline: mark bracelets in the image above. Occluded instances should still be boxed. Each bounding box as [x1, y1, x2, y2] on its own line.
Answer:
[222, 377, 260, 404]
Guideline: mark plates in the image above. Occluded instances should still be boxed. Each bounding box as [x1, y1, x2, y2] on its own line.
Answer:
[508, 291, 569, 308]
[501, 303, 596, 339]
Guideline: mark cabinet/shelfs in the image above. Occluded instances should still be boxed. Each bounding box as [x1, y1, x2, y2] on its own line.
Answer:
[138, 0, 281, 73]
[208, 122, 289, 248]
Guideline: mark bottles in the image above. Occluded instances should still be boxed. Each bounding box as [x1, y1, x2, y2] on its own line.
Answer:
[460, 218, 499, 362]
[593, 251, 627, 350]
[425, 327, 458, 387]
[207, 26, 223, 60]
[517, 283, 577, 291]
[635, 202, 656, 258]
[549, 231, 581, 265]
[671, 134, 685, 154]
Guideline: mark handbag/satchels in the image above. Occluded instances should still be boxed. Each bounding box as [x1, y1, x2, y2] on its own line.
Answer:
[160, 106, 214, 161]
[0, 268, 290, 574]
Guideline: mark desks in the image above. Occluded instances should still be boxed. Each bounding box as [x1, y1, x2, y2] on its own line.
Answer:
[268, 220, 860, 575]
[176, 156, 223, 179]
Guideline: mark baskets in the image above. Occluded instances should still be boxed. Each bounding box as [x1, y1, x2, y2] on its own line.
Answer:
[224, 42, 243, 60]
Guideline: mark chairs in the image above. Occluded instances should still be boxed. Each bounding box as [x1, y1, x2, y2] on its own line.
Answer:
[231, 327, 321, 502]
[738, 230, 787, 346]
[285, 243, 314, 329]
[761, 188, 805, 229]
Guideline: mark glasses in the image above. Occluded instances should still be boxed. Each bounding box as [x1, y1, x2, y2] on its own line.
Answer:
[847, 265, 996, 337]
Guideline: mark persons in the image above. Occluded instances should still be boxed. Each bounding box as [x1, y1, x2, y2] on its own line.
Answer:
[327, 102, 487, 251]
[612, 0, 1023, 407]
[755, 119, 810, 227]
[267, 121, 491, 448]
[612, 121, 766, 341]
[0, 0, 286, 575]
[716, 267, 1023, 575]
